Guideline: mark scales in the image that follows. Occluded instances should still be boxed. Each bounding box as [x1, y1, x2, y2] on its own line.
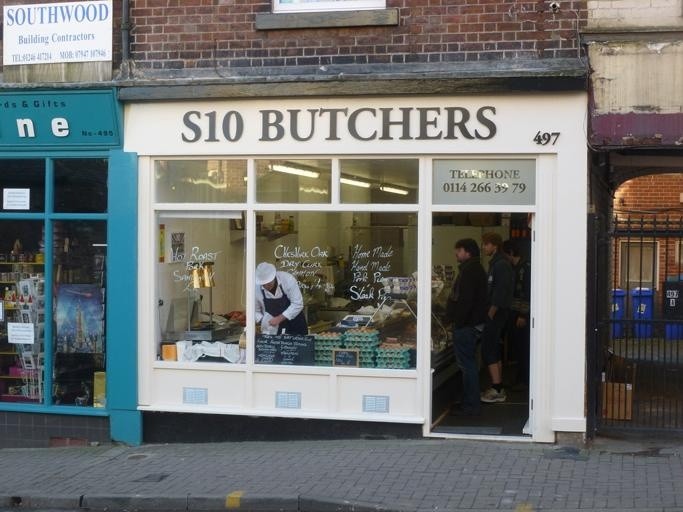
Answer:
[335, 312, 375, 329]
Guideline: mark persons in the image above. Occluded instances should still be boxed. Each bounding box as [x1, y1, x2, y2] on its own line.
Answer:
[255, 261, 309, 336]
[443, 231, 531, 409]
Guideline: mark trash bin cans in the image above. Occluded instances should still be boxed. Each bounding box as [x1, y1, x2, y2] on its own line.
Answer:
[631, 287, 654, 338]
[611, 288, 625, 340]
[664, 323, 683, 339]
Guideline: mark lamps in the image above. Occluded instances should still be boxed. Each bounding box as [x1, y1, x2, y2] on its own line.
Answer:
[268, 160, 410, 196]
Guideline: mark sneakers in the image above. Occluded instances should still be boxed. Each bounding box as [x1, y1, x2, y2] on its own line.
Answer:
[453, 405, 480, 416]
[480, 388, 508, 402]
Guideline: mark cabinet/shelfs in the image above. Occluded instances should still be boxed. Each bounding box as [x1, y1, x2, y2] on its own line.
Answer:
[366, 297, 459, 392]
[0, 261, 57, 402]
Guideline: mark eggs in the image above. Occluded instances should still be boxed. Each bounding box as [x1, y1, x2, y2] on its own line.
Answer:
[318, 327, 402, 351]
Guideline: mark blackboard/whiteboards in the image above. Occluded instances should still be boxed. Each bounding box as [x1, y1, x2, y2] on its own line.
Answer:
[333, 349, 360, 368]
[255, 334, 315, 367]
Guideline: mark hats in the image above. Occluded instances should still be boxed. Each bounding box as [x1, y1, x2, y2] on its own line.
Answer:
[256, 262, 277, 286]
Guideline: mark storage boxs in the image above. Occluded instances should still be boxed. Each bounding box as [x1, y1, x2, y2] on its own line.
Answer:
[599, 361, 637, 420]
